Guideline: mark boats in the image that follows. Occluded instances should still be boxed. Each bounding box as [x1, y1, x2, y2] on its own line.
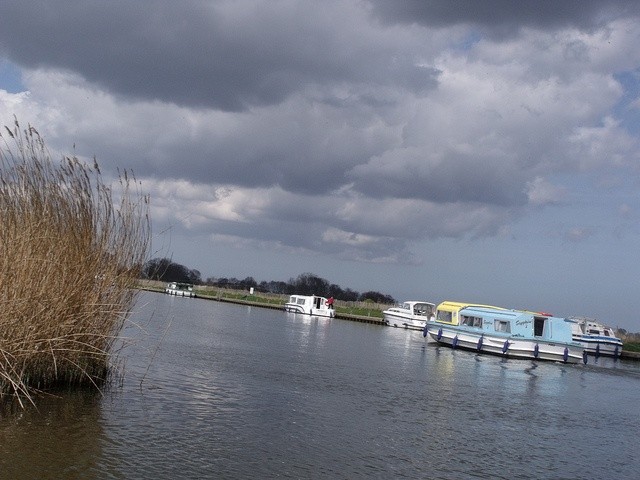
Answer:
[165, 282, 196, 297]
[382, 301, 436, 331]
[433, 301, 552, 326]
[423, 306, 588, 365]
[284, 294, 336, 318]
[561, 315, 624, 359]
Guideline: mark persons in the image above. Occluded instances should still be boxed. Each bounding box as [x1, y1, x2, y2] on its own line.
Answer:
[325, 297, 335, 309]
